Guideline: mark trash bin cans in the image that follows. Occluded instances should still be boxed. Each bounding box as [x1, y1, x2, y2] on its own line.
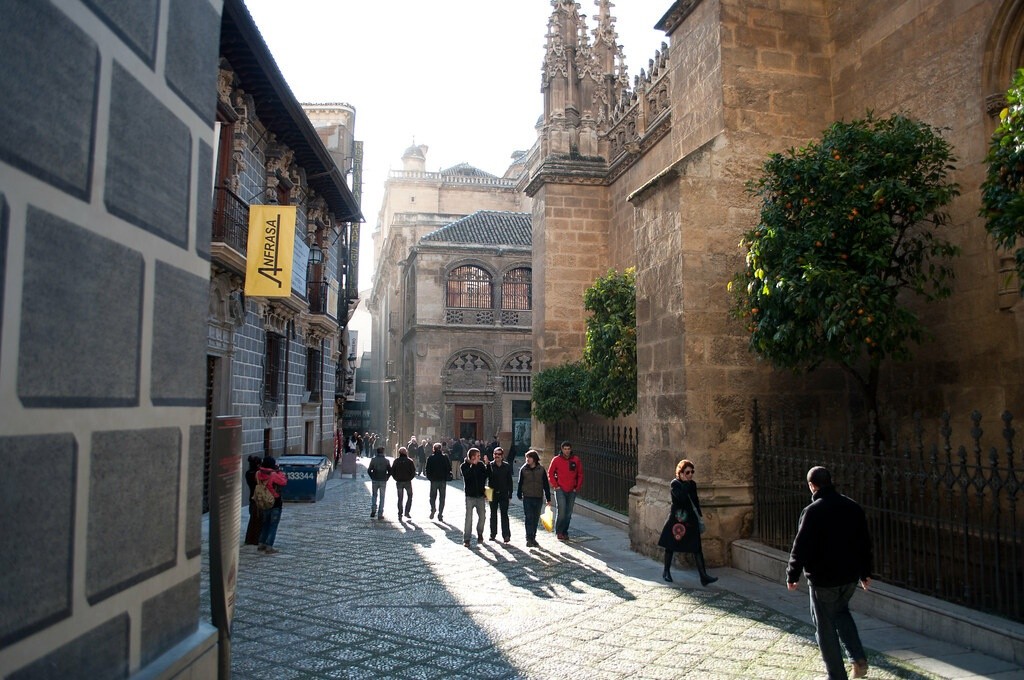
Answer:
[274, 453, 332, 503]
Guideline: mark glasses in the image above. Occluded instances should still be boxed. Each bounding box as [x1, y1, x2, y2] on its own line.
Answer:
[685, 470, 694, 475]
[494, 453, 502, 455]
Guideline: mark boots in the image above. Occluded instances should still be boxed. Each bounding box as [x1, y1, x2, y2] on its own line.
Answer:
[695, 554, 719, 586]
[662, 550, 673, 582]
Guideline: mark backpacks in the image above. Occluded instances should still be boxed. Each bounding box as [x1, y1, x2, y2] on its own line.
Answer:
[252, 471, 275, 509]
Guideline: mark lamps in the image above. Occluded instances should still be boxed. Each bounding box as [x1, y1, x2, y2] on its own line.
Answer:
[340, 353, 357, 375]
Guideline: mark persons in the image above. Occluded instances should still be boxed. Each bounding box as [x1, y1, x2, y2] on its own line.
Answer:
[392, 447, 416, 517]
[487, 446, 514, 542]
[658, 460, 718, 587]
[548, 441, 583, 539]
[517, 450, 552, 547]
[442, 436, 500, 480]
[368, 447, 392, 519]
[344, 431, 383, 457]
[460, 448, 493, 548]
[786, 466, 875, 680]
[254, 456, 288, 554]
[244, 455, 262, 546]
[407, 435, 433, 476]
[426, 443, 451, 521]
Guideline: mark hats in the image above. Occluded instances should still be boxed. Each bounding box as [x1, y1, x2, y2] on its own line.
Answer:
[248, 456, 260, 468]
[262, 457, 275, 468]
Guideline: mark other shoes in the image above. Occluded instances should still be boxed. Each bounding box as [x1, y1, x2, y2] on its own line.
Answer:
[563, 535, 569, 540]
[405, 512, 411, 518]
[398, 513, 403, 518]
[533, 541, 539, 547]
[853, 659, 867, 677]
[430, 513, 434, 519]
[465, 542, 470, 547]
[438, 515, 443, 521]
[527, 541, 533, 547]
[258, 545, 266, 551]
[504, 537, 510, 542]
[371, 513, 375, 517]
[266, 548, 279, 555]
[378, 516, 384, 520]
[478, 537, 484, 544]
[557, 534, 564, 541]
[489, 536, 495, 541]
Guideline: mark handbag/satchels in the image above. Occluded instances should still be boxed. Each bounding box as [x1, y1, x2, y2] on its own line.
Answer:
[446, 473, 453, 481]
[540, 505, 553, 532]
[485, 486, 494, 502]
[698, 518, 705, 533]
